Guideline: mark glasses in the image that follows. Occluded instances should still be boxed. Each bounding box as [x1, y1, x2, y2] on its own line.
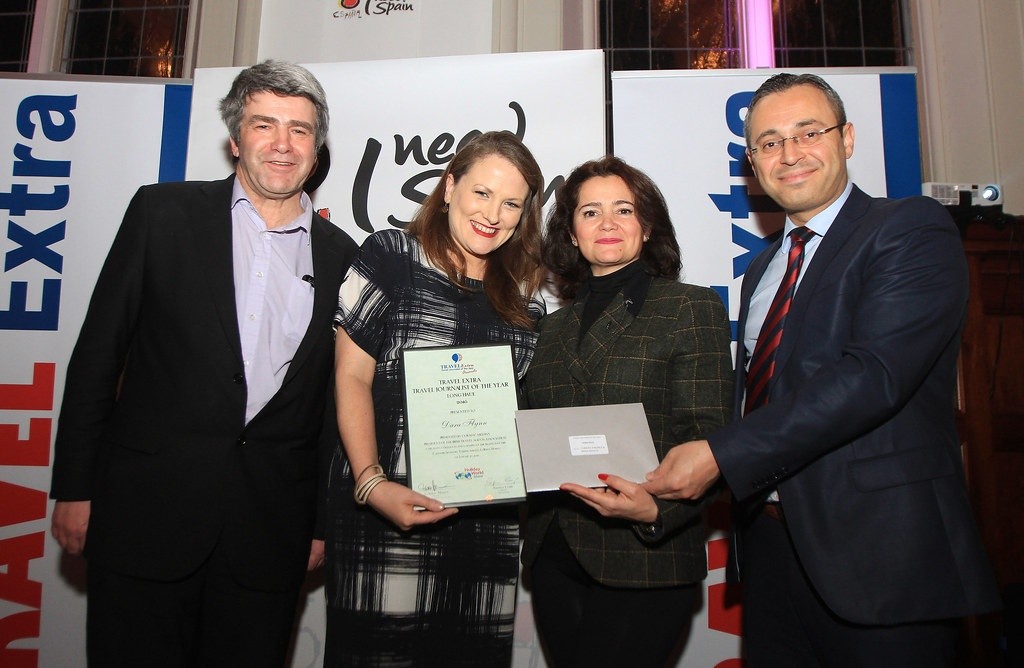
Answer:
[748, 122, 846, 157]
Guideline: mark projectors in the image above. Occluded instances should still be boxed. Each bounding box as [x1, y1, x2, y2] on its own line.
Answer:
[922, 182, 1003, 213]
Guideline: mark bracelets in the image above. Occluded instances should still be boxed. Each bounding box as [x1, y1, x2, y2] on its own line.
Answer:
[353, 464, 388, 505]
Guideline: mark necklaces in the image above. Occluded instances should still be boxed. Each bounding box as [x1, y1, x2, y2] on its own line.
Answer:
[445, 261, 486, 293]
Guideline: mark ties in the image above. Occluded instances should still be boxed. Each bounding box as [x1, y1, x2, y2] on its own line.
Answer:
[744, 226, 815, 417]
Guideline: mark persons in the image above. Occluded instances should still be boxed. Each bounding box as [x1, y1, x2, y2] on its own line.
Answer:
[323, 132, 545, 668]
[639, 73, 1004, 668]
[50, 62, 361, 668]
[517, 159, 734, 668]
